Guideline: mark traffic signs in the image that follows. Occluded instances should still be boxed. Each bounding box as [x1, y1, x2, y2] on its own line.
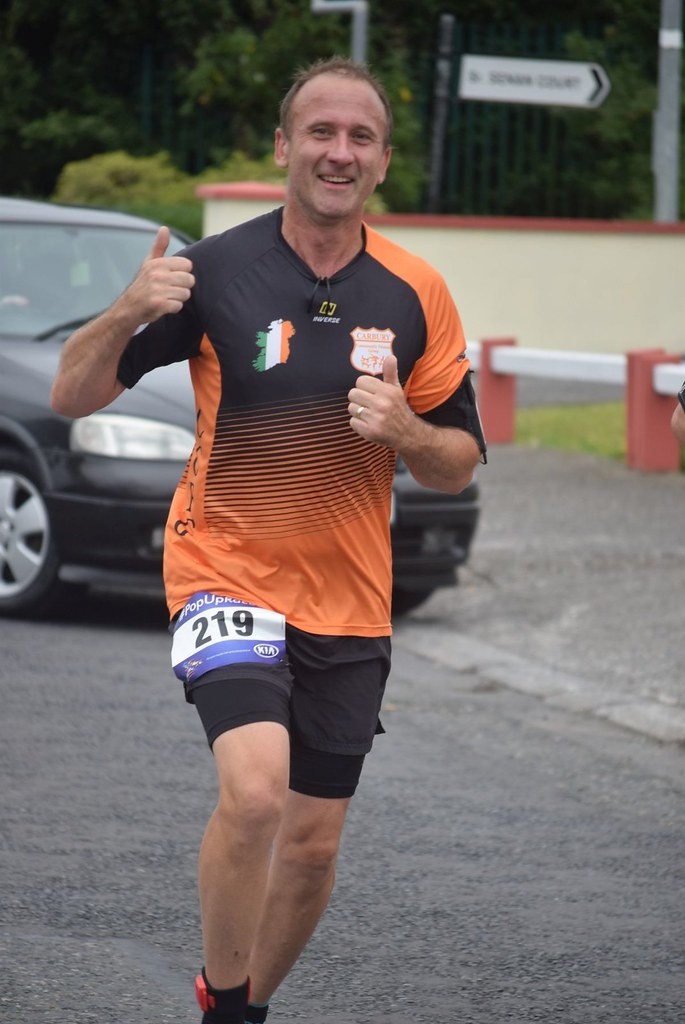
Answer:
[459, 53, 614, 111]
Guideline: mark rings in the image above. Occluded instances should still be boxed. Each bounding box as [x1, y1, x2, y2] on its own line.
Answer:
[355, 407, 363, 418]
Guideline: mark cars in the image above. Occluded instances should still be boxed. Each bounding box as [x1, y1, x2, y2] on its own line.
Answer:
[1, 196, 481, 623]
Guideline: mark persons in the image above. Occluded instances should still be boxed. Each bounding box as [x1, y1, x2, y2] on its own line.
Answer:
[50, 58, 487, 1024]
